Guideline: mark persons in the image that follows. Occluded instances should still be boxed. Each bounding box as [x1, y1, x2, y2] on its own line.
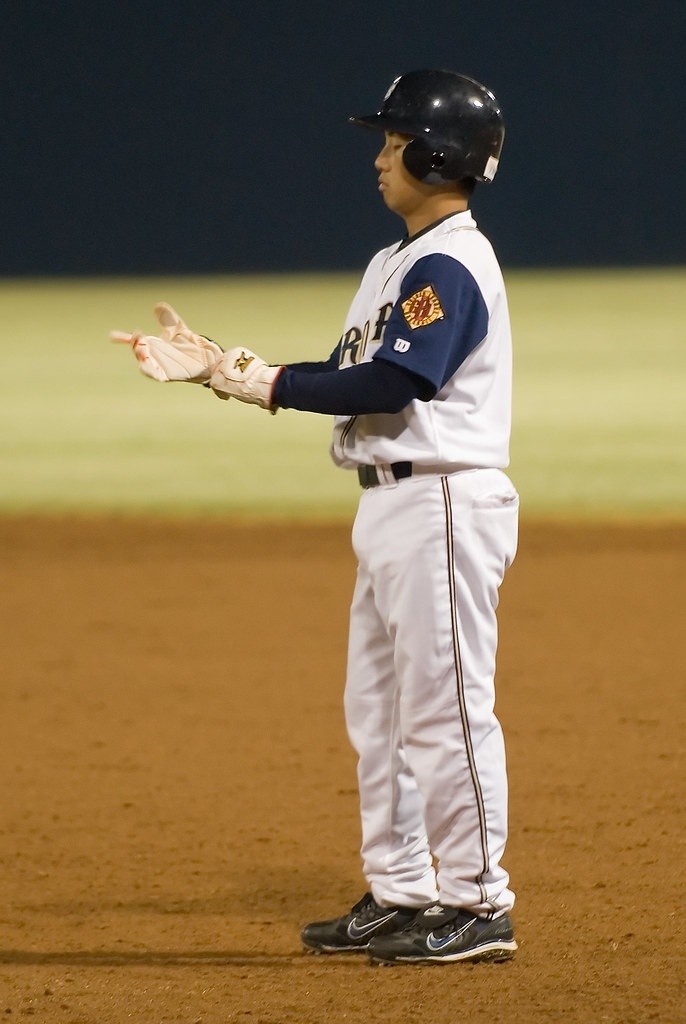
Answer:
[109, 69, 519, 966]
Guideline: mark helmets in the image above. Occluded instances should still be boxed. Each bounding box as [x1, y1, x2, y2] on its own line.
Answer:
[350, 68, 506, 184]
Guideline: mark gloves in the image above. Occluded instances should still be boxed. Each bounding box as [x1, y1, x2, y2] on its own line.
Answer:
[208, 346, 288, 415]
[112, 301, 231, 401]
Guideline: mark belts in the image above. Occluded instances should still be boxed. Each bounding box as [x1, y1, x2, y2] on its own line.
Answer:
[357, 461, 414, 489]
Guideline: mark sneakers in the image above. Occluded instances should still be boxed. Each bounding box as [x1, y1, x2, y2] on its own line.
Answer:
[299, 892, 422, 952]
[366, 900, 518, 966]
